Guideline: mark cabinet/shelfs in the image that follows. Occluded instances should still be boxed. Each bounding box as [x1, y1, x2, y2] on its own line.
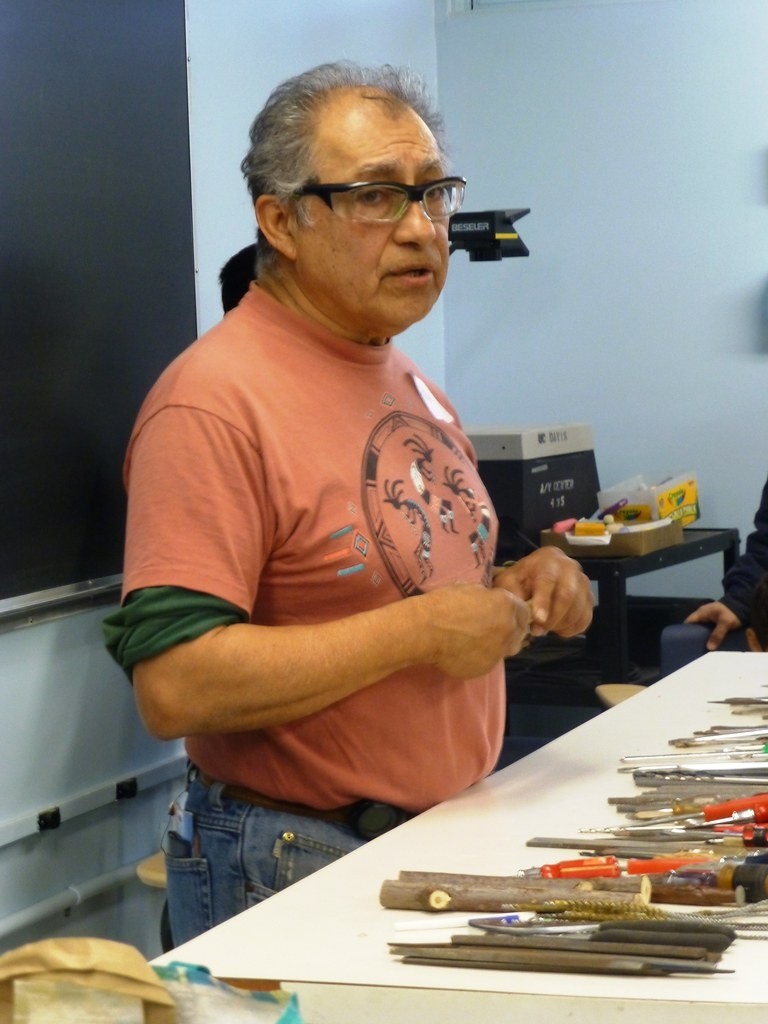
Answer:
[500, 524, 740, 738]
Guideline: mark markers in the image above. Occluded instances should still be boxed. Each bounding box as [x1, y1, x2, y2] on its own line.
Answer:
[75, 574, 121, 591]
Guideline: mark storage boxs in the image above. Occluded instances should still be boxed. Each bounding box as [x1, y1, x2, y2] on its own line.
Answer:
[465, 420, 602, 542]
[596, 470, 702, 527]
[538, 517, 685, 558]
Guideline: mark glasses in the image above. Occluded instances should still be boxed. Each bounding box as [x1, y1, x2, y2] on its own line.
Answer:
[294, 175, 467, 225]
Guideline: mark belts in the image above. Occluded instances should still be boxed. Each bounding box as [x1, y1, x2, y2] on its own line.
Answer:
[193, 767, 353, 825]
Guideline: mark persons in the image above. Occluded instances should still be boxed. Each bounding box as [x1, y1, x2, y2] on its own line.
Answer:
[658, 475, 768, 682]
[101, 57, 596, 947]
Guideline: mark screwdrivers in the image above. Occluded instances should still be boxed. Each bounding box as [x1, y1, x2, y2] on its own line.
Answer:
[509, 855, 622, 879]
[619, 743, 768, 763]
[681, 802, 768, 831]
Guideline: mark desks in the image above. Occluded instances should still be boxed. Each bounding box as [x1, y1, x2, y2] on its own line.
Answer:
[142, 650, 766, 1024]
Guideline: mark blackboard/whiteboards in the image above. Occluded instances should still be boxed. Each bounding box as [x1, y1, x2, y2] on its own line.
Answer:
[0, 1, 203, 634]
